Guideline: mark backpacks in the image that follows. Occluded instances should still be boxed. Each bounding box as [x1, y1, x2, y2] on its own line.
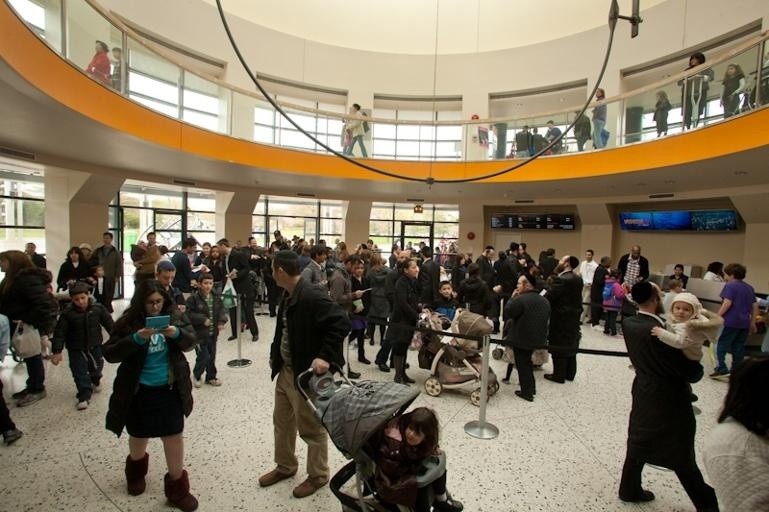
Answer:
[603, 283, 614, 300]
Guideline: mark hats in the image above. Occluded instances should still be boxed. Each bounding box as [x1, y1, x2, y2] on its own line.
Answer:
[80, 243, 91, 252]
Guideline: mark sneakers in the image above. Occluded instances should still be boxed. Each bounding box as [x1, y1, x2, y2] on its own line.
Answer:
[515, 389, 534, 402]
[227, 335, 237, 341]
[579, 317, 619, 336]
[709, 371, 731, 378]
[3, 384, 102, 446]
[543, 373, 565, 384]
[258, 466, 289, 487]
[192, 375, 203, 387]
[348, 338, 418, 384]
[292, 478, 330, 498]
[618, 491, 656, 503]
[252, 335, 258, 341]
[434, 492, 464, 511]
[204, 379, 221, 386]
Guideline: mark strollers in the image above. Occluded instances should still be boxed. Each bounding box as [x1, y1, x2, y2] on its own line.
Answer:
[618, 295, 637, 336]
[295, 362, 454, 512]
[415, 310, 500, 407]
[56, 275, 96, 312]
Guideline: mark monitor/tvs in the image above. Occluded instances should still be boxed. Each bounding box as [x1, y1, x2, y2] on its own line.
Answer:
[653, 210, 690, 231]
[546, 214, 576, 230]
[689, 208, 738, 232]
[491, 212, 518, 228]
[619, 210, 653, 231]
[517, 213, 546, 229]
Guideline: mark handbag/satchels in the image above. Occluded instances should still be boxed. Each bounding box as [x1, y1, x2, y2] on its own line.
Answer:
[592, 129, 609, 148]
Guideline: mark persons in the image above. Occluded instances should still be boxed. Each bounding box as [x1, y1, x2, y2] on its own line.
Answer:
[340, 104, 370, 159]
[709, 263, 758, 379]
[258, 250, 351, 499]
[653, 52, 768, 138]
[514, 87, 607, 159]
[51, 282, 115, 411]
[378, 407, 463, 511]
[84, 40, 130, 99]
[702, 350, 768, 512]
[0, 242, 60, 445]
[55, 230, 446, 385]
[429, 241, 724, 384]
[502, 272, 551, 402]
[622, 280, 719, 512]
[651, 291, 725, 402]
[105, 278, 198, 512]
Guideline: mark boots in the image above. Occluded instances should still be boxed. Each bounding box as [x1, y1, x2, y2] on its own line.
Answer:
[125, 451, 149, 496]
[164, 470, 198, 511]
